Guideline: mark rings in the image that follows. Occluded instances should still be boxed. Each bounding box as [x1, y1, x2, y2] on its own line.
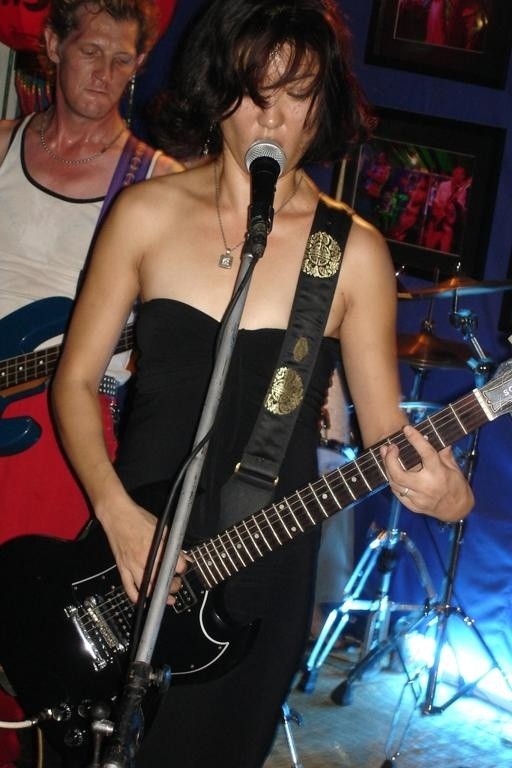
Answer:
[399, 485, 410, 498]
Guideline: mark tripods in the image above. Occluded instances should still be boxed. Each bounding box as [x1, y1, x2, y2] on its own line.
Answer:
[295, 319, 458, 695]
[330, 307, 511, 715]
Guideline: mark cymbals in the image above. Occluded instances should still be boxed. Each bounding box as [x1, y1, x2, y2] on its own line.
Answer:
[397, 274, 511, 301]
[396, 331, 489, 371]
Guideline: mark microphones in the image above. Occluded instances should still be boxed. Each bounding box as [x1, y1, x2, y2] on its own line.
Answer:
[243, 138, 289, 259]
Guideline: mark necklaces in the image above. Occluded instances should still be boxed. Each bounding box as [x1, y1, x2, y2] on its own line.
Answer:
[212, 155, 304, 269]
[37, 111, 128, 166]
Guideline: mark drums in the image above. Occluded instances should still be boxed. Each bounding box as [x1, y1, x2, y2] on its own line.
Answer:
[350, 405, 473, 478]
[312, 433, 359, 603]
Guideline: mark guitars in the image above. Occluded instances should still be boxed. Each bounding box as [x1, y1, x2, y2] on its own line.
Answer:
[0, 322, 137, 456]
[0, 350, 512, 719]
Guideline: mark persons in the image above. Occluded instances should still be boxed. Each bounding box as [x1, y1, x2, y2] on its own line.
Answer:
[362, 142, 474, 253]
[307, 367, 360, 652]
[1, 0, 189, 767]
[44, 1, 478, 768]
